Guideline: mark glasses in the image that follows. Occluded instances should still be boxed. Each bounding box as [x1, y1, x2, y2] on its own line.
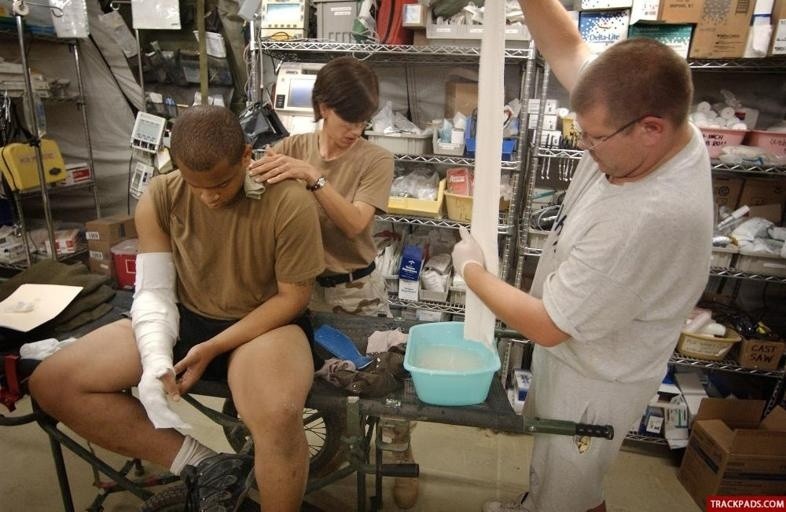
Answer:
[326, 109, 373, 130]
[573, 114, 663, 151]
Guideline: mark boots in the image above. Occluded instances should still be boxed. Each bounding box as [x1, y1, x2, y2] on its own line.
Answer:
[391, 427, 419, 509]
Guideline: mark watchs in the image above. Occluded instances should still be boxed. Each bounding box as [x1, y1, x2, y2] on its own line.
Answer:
[306, 176, 328, 192]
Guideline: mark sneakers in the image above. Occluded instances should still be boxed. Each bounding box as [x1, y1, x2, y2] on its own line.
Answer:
[481, 494, 530, 512]
[179, 452, 256, 512]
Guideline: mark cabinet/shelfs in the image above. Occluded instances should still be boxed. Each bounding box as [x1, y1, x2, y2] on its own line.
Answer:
[251, 1, 538, 398]
[501, 0, 786, 462]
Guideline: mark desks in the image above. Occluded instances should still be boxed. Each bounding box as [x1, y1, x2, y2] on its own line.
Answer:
[0, 288, 616, 509]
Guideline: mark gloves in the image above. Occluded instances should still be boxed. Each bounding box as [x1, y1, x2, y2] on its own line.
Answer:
[451, 225, 484, 278]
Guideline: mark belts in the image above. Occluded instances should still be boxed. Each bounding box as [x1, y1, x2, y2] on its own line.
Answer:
[316, 260, 375, 287]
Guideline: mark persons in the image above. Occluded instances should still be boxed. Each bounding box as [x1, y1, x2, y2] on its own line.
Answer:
[27, 105, 326, 512]
[247, 56, 419, 510]
[452, 0, 714, 512]
